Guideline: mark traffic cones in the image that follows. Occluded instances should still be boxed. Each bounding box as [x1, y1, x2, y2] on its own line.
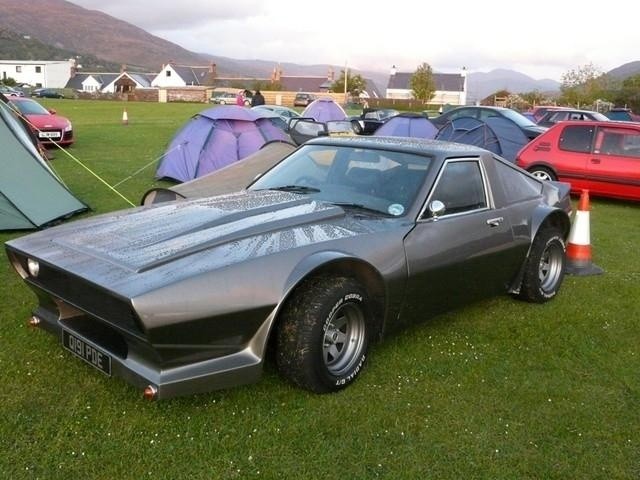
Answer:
[563, 185, 607, 278]
[121, 106, 129, 127]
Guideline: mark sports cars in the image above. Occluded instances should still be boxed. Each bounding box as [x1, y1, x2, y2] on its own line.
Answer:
[1, 132, 580, 405]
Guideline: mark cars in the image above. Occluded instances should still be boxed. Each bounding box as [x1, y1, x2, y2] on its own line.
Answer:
[252, 105, 304, 125]
[0, 84, 75, 149]
[348, 107, 400, 135]
[429, 105, 551, 140]
[208, 92, 252, 106]
[514, 104, 640, 208]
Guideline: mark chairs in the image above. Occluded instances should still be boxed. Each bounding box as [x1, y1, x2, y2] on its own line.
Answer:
[345, 167, 478, 212]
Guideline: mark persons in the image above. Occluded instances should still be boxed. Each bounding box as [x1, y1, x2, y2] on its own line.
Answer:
[250, 88, 265, 107]
[242, 90, 254, 106]
[236, 90, 246, 106]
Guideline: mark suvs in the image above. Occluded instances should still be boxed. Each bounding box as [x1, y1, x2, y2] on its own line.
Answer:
[293, 93, 317, 107]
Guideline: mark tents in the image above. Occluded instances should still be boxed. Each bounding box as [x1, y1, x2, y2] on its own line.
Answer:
[153, 96, 347, 185]
[373, 113, 529, 164]
[1, 89, 93, 234]
